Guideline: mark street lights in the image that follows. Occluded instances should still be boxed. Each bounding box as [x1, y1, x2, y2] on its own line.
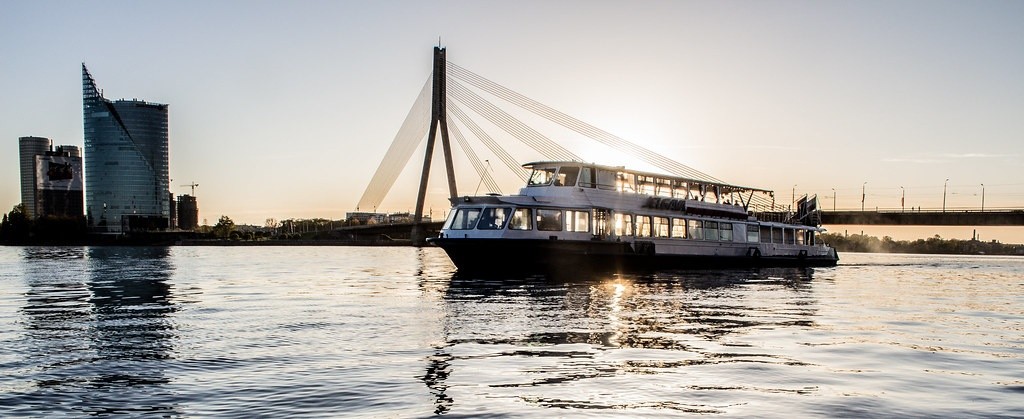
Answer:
[980, 183, 984, 212]
[943, 179, 949, 212]
[792, 184, 798, 211]
[831, 188, 836, 211]
[901, 186, 905, 213]
[861, 182, 867, 211]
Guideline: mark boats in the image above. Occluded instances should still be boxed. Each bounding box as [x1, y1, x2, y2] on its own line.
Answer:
[432, 160, 841, 280]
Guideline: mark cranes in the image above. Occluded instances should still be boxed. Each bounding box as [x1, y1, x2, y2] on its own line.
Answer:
[182, 181, 199, 196]
